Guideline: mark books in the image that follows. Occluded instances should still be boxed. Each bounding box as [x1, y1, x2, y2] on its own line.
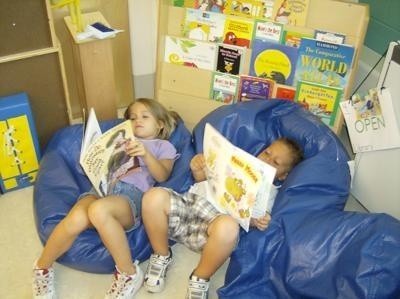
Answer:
[79, 106, 135, 198]
[296, 80, 344, 130]
[208, 70, 240, 106]
[202, 123, 278, 233]
[164, 0, 355, 103]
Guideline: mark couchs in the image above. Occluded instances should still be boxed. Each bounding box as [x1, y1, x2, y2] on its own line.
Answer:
[33, 115, 195, 275]
[191, 97, 354, 256]
[214, 209, 399, 297]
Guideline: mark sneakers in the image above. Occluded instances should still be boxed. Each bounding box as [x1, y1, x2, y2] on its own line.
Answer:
[32, 256, 56, 299]
[105, 246, 174, 299]
[186, 268, 210, 299]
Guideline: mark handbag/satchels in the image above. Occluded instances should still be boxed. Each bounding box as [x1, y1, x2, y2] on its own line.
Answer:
[339, 41, 400, 154]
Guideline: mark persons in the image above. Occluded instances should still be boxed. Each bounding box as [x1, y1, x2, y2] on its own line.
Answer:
[31, 98, 183, 299]
[140, 137, 304, 299]
